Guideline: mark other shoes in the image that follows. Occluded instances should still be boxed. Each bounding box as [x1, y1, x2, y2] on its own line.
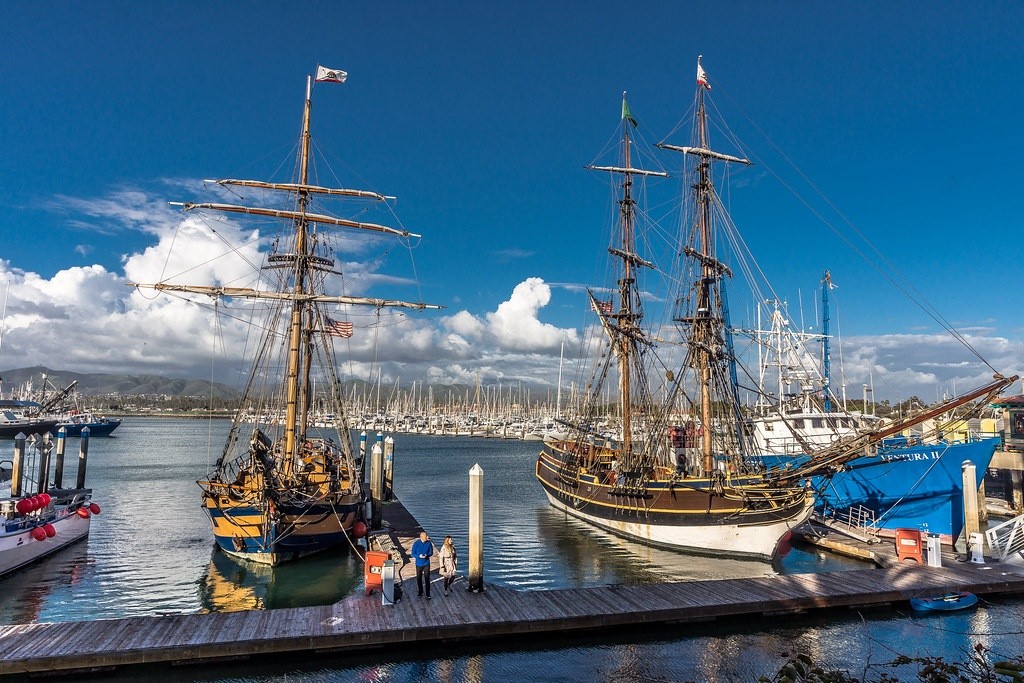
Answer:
[418, 593, 422, 597]
[444, 590, 448, 596]
[448, 585, 452, 591]
[425, 596, 431, 599]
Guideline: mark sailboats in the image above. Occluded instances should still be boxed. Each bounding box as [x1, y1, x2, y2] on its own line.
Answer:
[194, 547, 357, 615]
[548, 504, 778, 583]
[121, 70, 447, 567]
[229, 50, 1019, 560]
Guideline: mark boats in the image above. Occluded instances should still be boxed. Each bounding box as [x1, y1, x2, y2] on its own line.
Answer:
[0, 372, 125, 436]
[0, 421, 100, 577]
[910, 590, 979, 612]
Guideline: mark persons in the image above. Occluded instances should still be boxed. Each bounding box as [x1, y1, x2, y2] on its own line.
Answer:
[439, 535, 458, 596]
[412, 531, 433, 599]
